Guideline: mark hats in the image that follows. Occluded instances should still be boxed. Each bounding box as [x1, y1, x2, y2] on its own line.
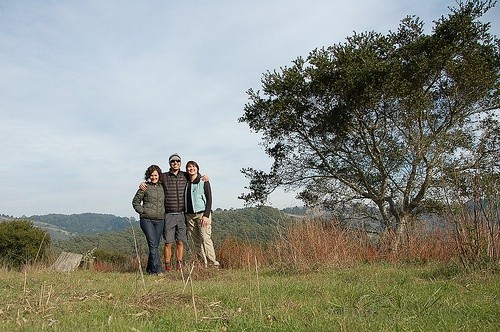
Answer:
[169, 153, 181, 162]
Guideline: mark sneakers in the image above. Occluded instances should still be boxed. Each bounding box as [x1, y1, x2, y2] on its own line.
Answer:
[177, 266, 183, 271]
[165, 266, 171, 270]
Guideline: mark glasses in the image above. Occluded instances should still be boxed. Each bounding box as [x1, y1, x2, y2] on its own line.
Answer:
[171, 160, 181, 162]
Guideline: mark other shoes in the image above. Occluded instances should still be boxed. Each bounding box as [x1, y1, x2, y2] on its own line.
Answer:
[157, 272, 163, 276]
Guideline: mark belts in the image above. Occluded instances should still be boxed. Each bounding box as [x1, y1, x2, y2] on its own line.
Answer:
[167, 212, 184, 214]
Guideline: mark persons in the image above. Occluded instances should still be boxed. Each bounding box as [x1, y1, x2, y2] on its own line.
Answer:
[138, 153, 210, 274]
[183, 160, 221, 272]
[132, 164, 167, 274]
